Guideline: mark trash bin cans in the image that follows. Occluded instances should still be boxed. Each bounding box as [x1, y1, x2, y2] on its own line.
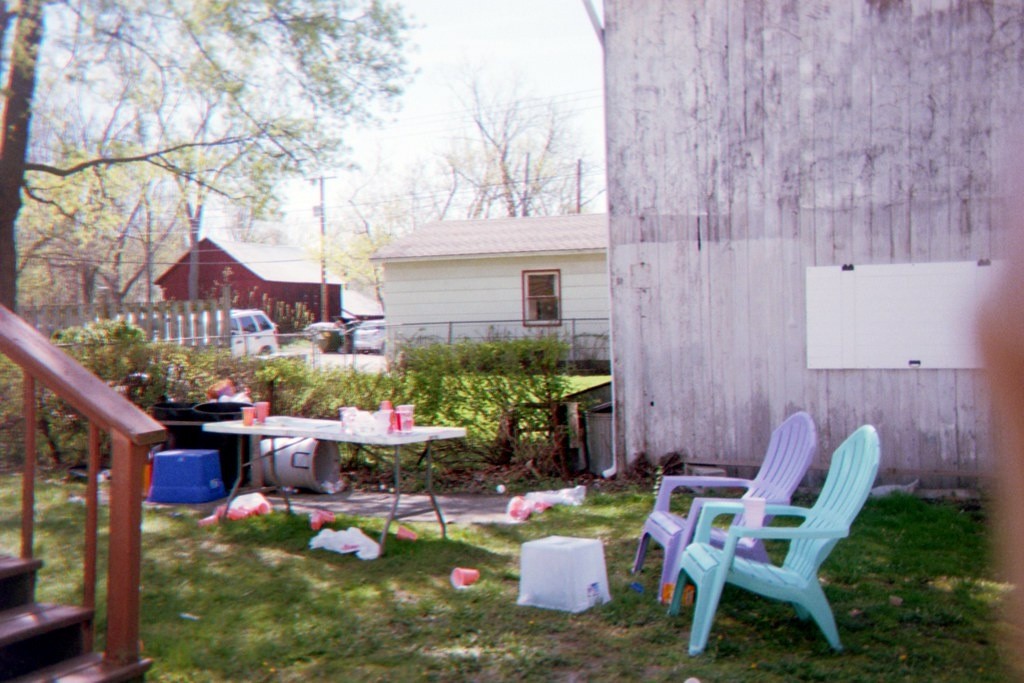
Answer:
[191, 401, 252, 490]
[260, 437, 341, 494]
[151, 402, 199, 452]
[317, 330, 340, 353]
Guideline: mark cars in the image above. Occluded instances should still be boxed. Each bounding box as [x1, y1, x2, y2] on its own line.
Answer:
[352, 318, 386, 354]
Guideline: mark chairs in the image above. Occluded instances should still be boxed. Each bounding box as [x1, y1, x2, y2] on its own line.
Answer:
[669, 424, 880, 657]
[632, 412, 817, 604]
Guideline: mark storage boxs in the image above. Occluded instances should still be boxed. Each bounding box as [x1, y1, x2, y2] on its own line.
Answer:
[660, 581, 694, 609]
[517, 535, 612, 614]
[146, 449, 226, 505]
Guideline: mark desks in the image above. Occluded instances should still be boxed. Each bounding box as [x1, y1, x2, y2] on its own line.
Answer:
[203, 417, 467, 558]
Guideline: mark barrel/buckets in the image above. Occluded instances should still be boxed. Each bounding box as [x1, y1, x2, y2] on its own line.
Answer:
[192, 402, 254, 490]
[150, 402, 198, 450]
[260, 436, 340, 494]
[585, 412, 613, 476]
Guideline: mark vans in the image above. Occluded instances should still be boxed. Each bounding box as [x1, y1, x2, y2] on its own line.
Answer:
[154, 306, 282, 356]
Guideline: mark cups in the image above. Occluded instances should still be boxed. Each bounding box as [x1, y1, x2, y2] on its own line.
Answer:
[255, 402, 270, 423]
[380, 401, 414, 432]
[310, 509, 334, 530]
[396, 524, 417, 542]
[243, 407, 257, 427]
[451, 567, 480, 588]
[197, 513, 219, 528]
[339, 407, 348, 420]
